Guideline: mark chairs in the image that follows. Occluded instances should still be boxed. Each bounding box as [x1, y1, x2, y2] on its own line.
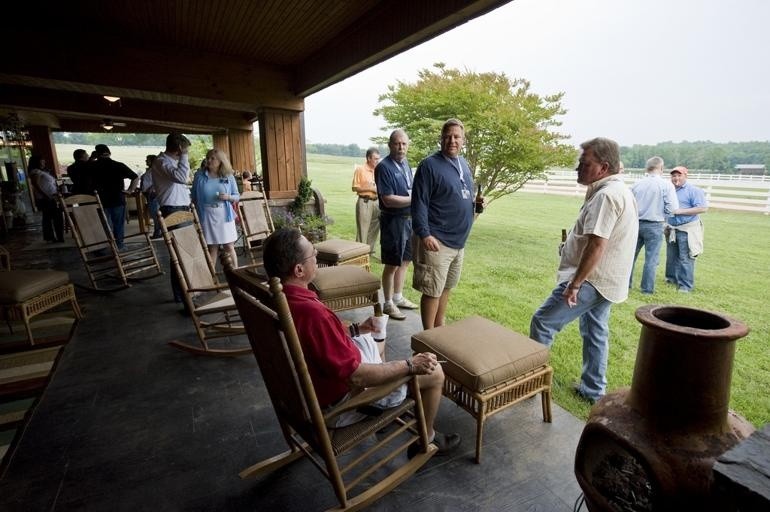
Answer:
[236, 186, 276, 274]
[219, 251, 440, 512]
[156, 203, 264, 356]
[58, 190, 166, 294]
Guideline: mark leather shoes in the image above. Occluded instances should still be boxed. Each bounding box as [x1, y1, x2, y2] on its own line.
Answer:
[383, 302, 407, 320]
[407, 430, 463, 459]
[392, 297, 419, 309]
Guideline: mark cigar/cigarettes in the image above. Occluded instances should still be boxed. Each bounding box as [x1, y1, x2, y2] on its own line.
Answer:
[435, 360, 449, 365]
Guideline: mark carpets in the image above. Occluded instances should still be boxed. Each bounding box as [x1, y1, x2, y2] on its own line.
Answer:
[0, 301, 88, 483]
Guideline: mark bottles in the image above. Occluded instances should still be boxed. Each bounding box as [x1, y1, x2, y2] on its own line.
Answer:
[474, 183, 485, 215]
[557, 228, 570, 263]
[216, 179, 227, 209]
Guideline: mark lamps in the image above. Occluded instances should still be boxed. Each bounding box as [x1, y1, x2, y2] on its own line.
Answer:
[103, 119, 113, 130]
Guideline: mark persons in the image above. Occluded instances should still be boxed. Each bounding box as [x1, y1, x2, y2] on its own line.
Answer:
[28, 153, 65, 243]
[67, 149, 98, 206]
[261, 226, 462, 460]
[664, 166, 709, 291]
[151, 134, 201, 302]
[76, 144, 139, 257]
[191, 149, 241, 271]
[627, 156, 681, 294]
[373, 128, 420, 321]
[528, 137, 639, 406]
[242, 170, 257, 192]
[618, 161, 624, 175]
[140, 155, 164, 239]
[351, 146, 382, 262]
[411, 117, 488, 330]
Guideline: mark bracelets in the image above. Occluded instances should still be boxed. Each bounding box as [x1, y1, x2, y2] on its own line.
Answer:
[349, 322, 360, 338]
[567, 283, 581, 290]
[405, 358, 413, 376]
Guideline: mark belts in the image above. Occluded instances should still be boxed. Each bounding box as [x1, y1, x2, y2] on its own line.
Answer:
[360, 195, 376, 201]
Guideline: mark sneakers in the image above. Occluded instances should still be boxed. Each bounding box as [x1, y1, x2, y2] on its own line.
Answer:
[571, 382, 595, 406]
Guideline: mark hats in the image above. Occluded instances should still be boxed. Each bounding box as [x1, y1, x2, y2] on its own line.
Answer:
[670, 166, 688, 175]
[95, 143, 111, 156]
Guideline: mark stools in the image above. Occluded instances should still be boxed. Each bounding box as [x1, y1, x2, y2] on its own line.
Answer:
[308, 265, 382, 317]
[0, 271, 84, 347]
[411, 313, 553, 464]
[312, 239, 371, 273]
